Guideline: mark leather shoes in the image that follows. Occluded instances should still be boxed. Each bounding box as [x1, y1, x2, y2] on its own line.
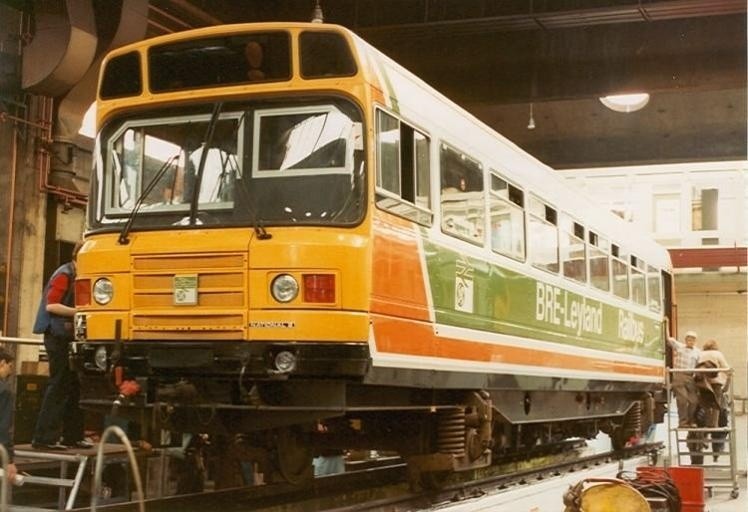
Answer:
[32, 438, 68, 450]
[65, 440, 95, 449]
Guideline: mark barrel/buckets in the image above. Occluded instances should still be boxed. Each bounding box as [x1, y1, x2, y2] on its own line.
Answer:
[636, 466, 705, 512]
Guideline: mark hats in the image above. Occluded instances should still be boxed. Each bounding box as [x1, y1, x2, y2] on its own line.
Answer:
[684, 332, 696, 340]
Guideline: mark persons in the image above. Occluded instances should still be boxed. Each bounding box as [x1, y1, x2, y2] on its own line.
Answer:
[29, 240, 93, 451]
[686, 339, 734, 466]
[663, 316, 700, 427]
[0, 345, 19, 484]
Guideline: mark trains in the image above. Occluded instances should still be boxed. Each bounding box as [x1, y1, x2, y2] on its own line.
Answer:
[68, 21, 681, 502]
